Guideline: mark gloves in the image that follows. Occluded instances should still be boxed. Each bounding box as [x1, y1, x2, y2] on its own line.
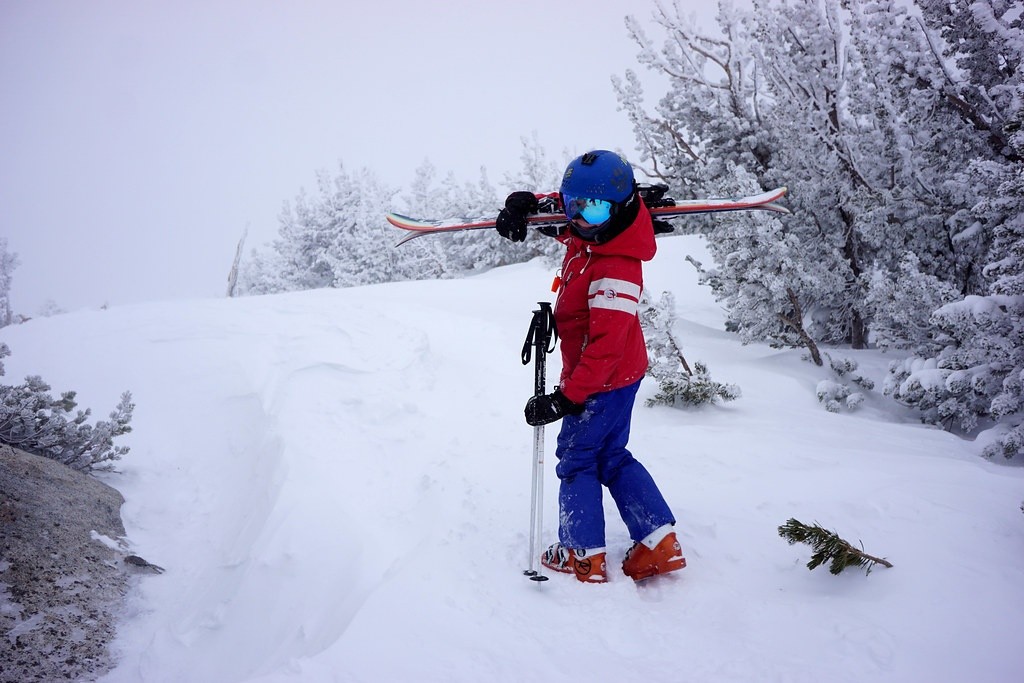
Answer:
[524, 389, 580, 426]
[496, 191, 539, 242]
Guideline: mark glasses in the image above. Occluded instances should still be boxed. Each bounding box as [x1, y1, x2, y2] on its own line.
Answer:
[561, 193, 613, 224]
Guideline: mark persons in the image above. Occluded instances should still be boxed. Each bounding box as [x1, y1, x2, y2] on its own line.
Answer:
[495, 150, 686, 583]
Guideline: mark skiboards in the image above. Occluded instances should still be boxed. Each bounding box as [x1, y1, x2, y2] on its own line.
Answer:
[386, 186, 790, 250]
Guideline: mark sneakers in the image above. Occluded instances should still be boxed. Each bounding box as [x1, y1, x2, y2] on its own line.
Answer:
[622, 523, 687, 582]
[541, 542, 607, 583]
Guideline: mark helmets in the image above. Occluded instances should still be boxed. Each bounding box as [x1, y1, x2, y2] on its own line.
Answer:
[559, 150, 636, 239]
